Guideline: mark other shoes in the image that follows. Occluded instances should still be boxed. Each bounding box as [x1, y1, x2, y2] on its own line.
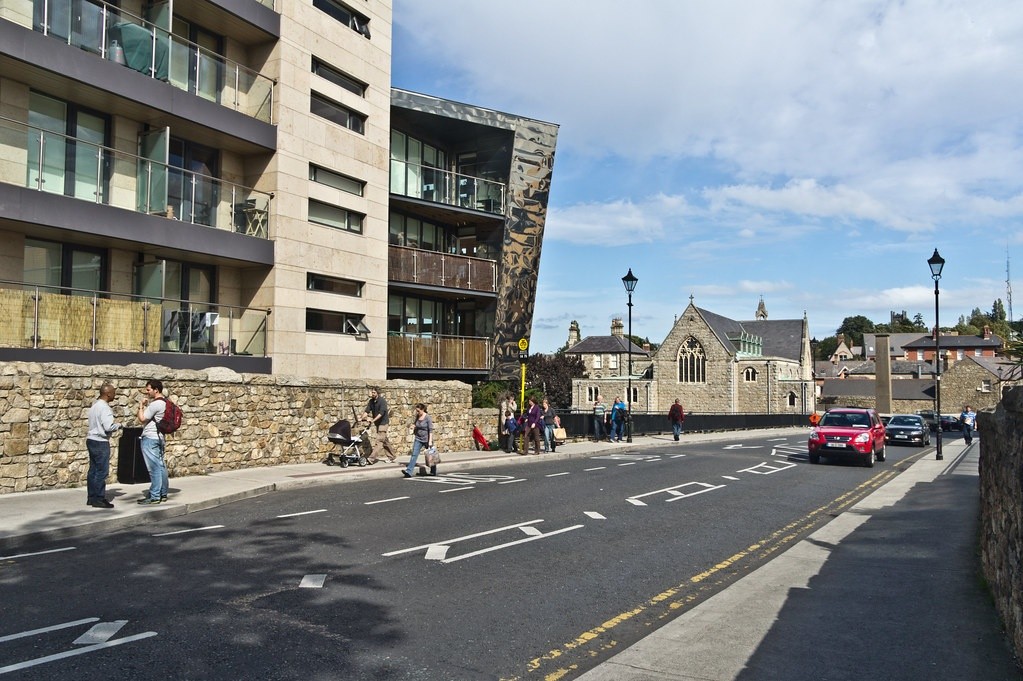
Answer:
[384, 457, 398, 463]
[513, 447, 517, 452]
[674, 436, 679, 441]
[552, 448, 555, 452]
[617, 439, 623, 443]
[506, 450, 512, 453]
[610, 439, 614, 443]
[521, 452, 527, 455]
[430, 473, 436, 476]
[401, 470, 412, 477]
[544, 451, 549, 453]
[532, 452, 539, 455]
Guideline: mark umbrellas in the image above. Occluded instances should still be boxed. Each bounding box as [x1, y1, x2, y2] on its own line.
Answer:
[472, 424, 491, 450]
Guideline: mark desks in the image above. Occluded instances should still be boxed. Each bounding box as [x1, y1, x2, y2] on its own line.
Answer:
[243, 208, 268, 237]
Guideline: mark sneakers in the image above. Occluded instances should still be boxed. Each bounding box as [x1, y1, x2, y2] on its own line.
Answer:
[136, 494, 161, 504]
[87, 500, 92, 505]
[92, 499, 114, 508]
[160, 495, 167, 501]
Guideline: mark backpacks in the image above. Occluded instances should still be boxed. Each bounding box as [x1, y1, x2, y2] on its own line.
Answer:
[151, 396, 183, 440]
[671, 404, 681, 418]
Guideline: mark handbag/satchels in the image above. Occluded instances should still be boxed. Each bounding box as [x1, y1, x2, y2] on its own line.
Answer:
[387, 408, 394, 418]
[552, 426, 567, 443]
[424, 445, 441, 468]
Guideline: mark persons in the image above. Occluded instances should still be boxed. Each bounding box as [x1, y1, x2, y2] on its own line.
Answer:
[520, 397, 540, 456]
[609, 396, 626, 444]
[402, 403, 437, 477]
[138, 379, 169, 504]
[360, 387, 397, 465]
[502, 410, 519, 454]
[539, 399, 560, 454]
[961, 404, 977, 447]
[86, 385, 122, 509]
[667, 398, 684, 442]
[593, 395, 611, 442]
[499, 394, 518, 451]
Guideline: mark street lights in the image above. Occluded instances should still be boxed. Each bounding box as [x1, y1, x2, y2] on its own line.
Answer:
[926, 248, 946, 461]
[621, 268, 639, 443]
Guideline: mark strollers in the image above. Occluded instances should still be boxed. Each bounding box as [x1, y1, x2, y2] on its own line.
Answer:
[326, 419, 372, 469]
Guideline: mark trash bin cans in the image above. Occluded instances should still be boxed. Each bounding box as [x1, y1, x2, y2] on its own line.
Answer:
[117, 427, 152, 484]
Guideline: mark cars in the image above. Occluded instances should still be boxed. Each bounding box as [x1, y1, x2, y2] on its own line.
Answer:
[807, 406, 887, 468]
[937, 415, 961, 433]
[878, 410, 935, 447]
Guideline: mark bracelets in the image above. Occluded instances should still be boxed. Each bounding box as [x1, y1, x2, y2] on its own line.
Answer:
[604, 418, 607, 419]
